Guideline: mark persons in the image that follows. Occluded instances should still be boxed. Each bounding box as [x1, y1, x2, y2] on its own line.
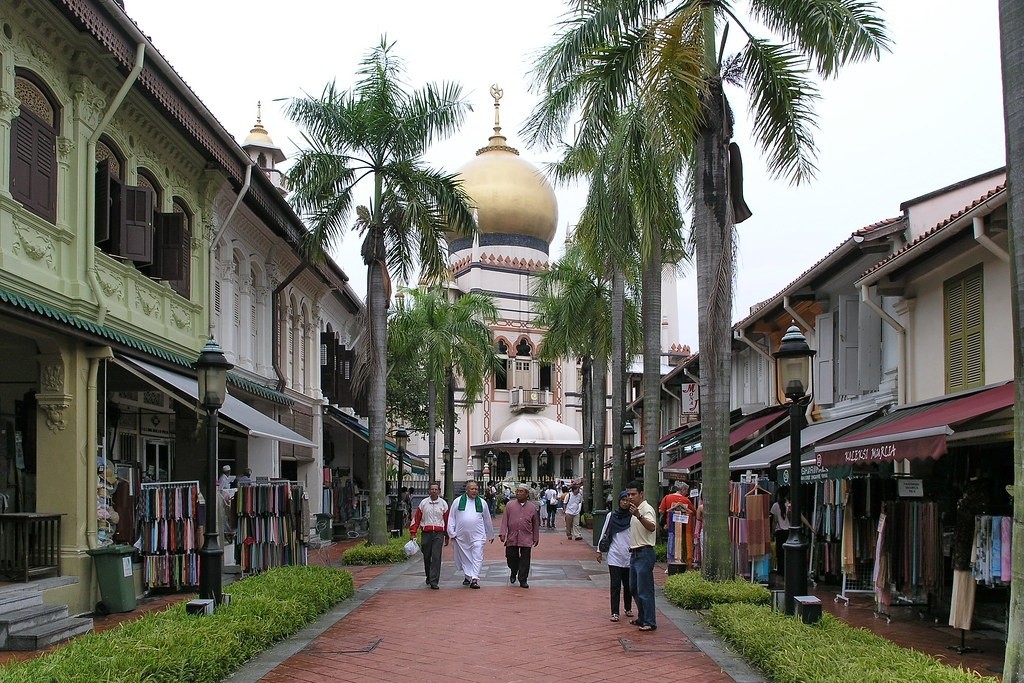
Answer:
[219, 464, 236, 489]
[694, 490, 703, 561]
[447, 482, 495, 589]
[485, 481, 496, 518]
[498, 483, 541, 586]
[238, 468, 252, 486]
[503, 484, 510, 505]
[409, 482, 450, 589]
[563, 485, 583, 540]
[402, 486, 414, 521]
[769, 486, 816, 572]
[659, 482, 697, 573]
[528, 481, 569, 529]
[596, 490, 634, 622]
[626, 481, 657, 631]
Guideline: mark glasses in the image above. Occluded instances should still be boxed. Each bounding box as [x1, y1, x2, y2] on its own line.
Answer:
[470, 490, 478, 493]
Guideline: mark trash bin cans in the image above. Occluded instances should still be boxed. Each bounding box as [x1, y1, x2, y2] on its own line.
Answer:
[313, 512, 333, 542]
[86, 543, 138, 616]
[592, 510, 610, 547]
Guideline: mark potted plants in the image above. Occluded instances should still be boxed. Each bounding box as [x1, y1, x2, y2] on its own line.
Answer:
[584, 512, 593, 529]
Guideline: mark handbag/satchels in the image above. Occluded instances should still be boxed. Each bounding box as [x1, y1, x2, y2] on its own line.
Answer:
[539, 500, 544, 507]
[404, 538, 419, 558]
[787, 505, 792, 522]
[550, 498, 558, 505]
[534, 496, 539, 511]
[599, 536, 609, 551]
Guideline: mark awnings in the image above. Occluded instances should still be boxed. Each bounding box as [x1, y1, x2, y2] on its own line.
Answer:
[336, 413, 425, 468]
[119, 353, 319, 449]
[626, 408, 869, 486]
[815, 384, 1016, 468]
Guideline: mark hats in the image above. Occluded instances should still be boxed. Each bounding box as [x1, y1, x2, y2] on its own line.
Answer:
[504, 483, 510, 488]
[223, 465, 231, 472]
[518, 483, 530, 491]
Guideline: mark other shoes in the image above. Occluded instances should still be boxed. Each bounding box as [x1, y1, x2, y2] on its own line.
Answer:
[470, 583, 480, 589]
[575, 536, 583, 540]
[426, 578, 430, 584]
[639, 625, 656, 631]
[431, 585, 439, 590]
[547, 525, 551, 528]
[552, 525, 557, 528]
[510, 575, 516, 583]
[568, 536, 572, 540]
[630, 620, 639, 625]
[610, 614, 620, 622]
[463, 578, 470, 585]
[624, 609, 634, 617]
[520, 582, 529, 588]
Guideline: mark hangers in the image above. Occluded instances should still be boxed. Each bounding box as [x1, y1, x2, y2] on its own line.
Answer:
[666, 500, 694, 515]
[744, 484, 772, 497]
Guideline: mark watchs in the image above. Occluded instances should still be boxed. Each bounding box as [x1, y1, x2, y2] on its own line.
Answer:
[637, 515, 643, 519]
